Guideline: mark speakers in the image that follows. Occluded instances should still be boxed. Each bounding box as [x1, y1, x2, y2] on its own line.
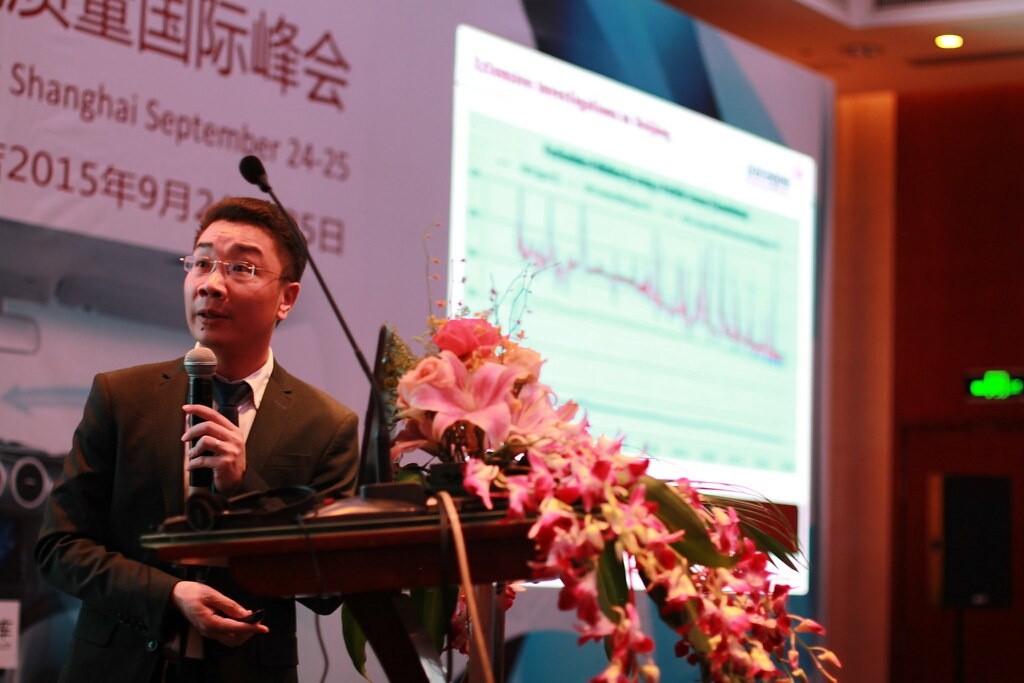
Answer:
[941, 475, 1012, 608]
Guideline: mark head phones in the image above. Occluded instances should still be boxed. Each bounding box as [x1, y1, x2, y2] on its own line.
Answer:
[186, 484, 316, 534]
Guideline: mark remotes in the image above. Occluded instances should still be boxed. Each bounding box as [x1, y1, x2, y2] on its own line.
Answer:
[238, 609, 266, 624]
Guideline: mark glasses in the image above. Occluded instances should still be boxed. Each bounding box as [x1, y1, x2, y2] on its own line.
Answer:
[179, 254, 290, 282]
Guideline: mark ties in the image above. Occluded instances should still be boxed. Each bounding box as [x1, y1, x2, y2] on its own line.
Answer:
[210, 376, 252, 493]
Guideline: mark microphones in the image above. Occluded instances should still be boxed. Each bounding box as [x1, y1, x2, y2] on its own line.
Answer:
[238, 155, 385, 407]
[184, 347, 217, 486]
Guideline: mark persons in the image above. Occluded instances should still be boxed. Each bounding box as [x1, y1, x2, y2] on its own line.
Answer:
[34, 197, 361, 683]
